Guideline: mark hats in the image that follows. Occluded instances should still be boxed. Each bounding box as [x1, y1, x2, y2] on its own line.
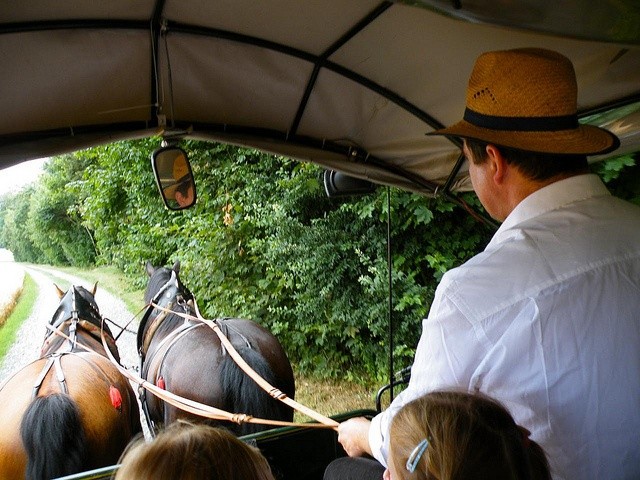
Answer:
[425, 47, 621, 156]
[162, 153, 193, 200]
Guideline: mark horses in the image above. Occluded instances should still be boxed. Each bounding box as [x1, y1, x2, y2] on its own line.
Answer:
[137, 259, 295, 439]
[0, 279, 144, 480]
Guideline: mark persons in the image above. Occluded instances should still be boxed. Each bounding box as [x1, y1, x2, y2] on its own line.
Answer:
[114, 420, 274, 480]
[162, 154, 194, 208]
[383, 392, 551, 480]
[323, 48, 640, 480]
